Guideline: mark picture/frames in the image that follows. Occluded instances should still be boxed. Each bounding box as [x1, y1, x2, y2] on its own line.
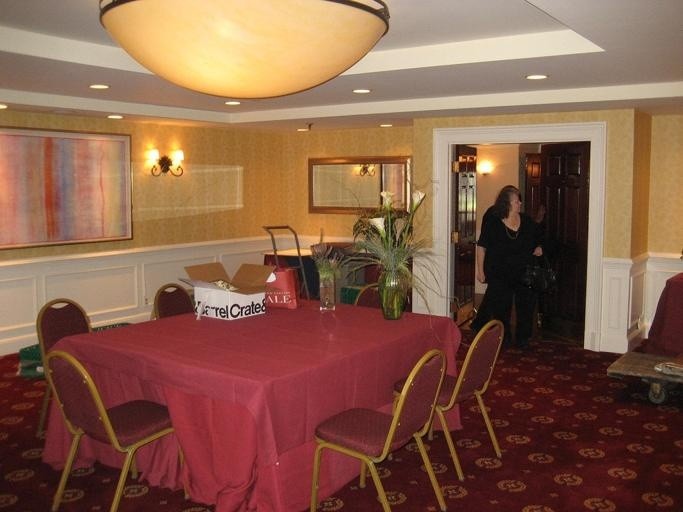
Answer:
[1, 126, 133, 250]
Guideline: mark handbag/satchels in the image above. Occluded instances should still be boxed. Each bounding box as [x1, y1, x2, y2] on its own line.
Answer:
[527, 252, 557, 294]
[264, 267, 300, 310]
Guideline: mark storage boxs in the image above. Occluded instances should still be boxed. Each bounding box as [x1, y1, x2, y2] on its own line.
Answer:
[177, 261, 278, 322]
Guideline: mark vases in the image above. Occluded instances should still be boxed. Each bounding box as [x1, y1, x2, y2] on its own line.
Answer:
[377, 271, 408, 320]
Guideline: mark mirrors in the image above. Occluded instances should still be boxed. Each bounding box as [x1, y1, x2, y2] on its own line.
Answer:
[307, 155, 413, 219]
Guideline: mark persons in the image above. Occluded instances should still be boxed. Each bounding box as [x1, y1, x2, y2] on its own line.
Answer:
[479, 184, 547, 233]
[463, 186, 545, 353]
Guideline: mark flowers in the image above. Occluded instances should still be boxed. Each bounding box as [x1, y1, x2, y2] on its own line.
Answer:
[343, 189, 445, 319]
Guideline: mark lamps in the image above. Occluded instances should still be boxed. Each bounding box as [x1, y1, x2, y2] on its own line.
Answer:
[148, 149, 184, 177]
[97, 1, 389, 101]
